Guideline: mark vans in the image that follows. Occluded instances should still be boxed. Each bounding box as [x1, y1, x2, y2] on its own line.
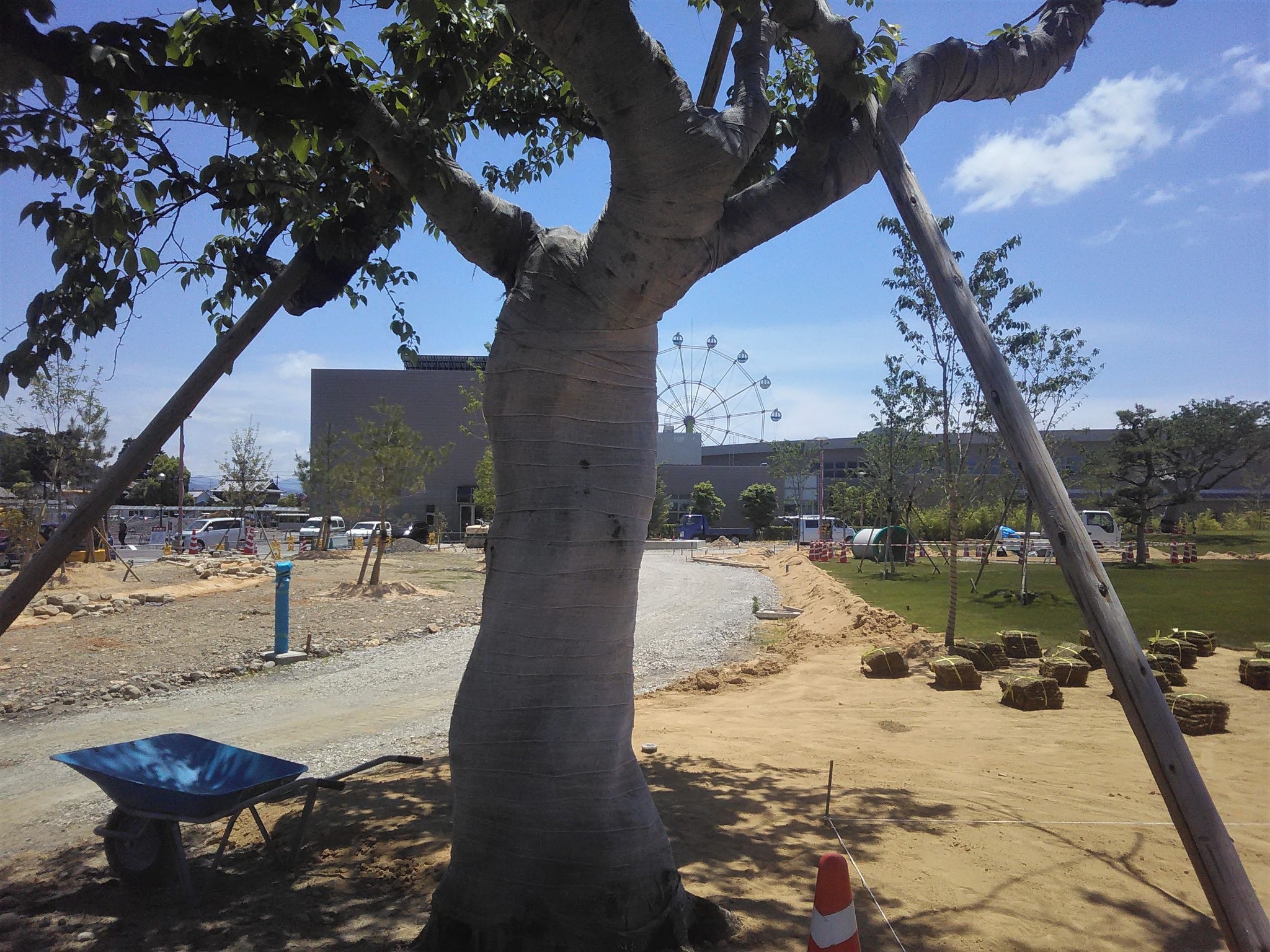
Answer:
[798, 515, 856, 546]
[171, 517, 246, 553]
[299, 516, 346, 544]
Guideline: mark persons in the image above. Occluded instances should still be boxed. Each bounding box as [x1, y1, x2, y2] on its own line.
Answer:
[118, 517, 127, 549]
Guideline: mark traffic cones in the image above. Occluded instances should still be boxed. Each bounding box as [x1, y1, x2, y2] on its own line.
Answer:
[804, 852, 862, 952]
[975, 538, 982, 557]
[961, 538, 973, 558]
[908, 541, 916, 564]
[918, 538, 927, 557]
[241, 527, 258, 555]
[1015, 541, 1028, 566]
[253, 543, 259, 557]
[302, 536, 310, 551]
[836, 540, 851, 563]
[297, 541, 304, 554]
[188, 528, 200, 555]
[976, 541, 992, 566]
[1125, 519, 1198, 564]
[808, 540, 818, 561]
[940, 538, 950, 557]
[814, 538, 838, 562]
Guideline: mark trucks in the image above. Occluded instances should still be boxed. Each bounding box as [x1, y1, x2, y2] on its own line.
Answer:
[1002, 509, 1122, 558]
[675, 514, 753, 545]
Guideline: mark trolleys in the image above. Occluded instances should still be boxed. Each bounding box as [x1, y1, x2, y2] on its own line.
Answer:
[48, 731, 423, 909]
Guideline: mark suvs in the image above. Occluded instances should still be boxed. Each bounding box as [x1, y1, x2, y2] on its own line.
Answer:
[346, 521, 392, 545]
[391, 521, 430, 544]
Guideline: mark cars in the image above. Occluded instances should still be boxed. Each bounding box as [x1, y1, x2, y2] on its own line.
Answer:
[0, 523, 114, 569]
